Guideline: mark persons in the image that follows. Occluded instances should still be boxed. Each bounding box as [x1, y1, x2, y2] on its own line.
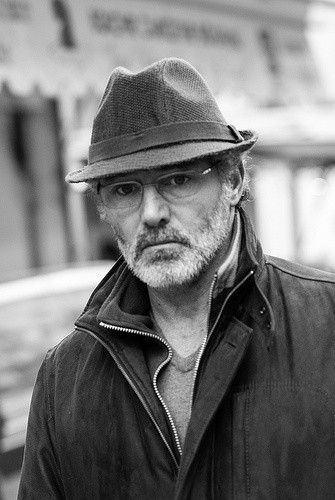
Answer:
[16, 58, 334, 500]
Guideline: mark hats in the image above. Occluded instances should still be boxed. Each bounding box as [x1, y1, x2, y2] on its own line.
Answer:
[64, 57, 259, 183]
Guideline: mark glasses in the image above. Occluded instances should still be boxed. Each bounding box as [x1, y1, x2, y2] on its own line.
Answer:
[97, 159, 222, 209]
